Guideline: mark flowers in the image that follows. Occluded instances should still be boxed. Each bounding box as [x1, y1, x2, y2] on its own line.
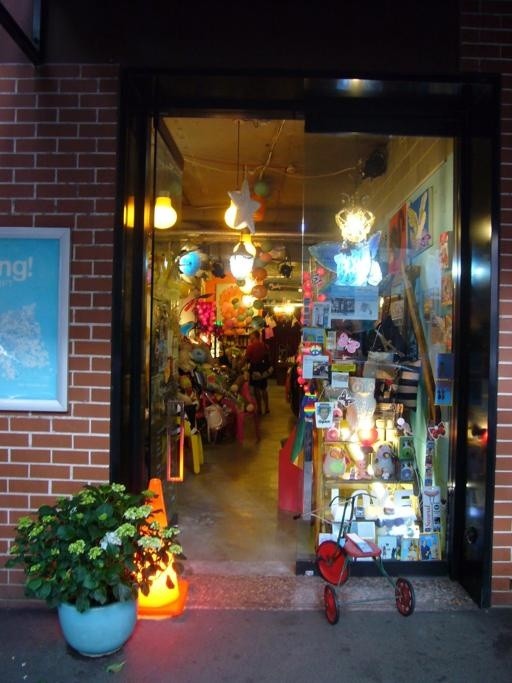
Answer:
[8, 480, 184, 610]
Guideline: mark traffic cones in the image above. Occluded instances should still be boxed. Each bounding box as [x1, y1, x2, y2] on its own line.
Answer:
[130, 479, 189, 615]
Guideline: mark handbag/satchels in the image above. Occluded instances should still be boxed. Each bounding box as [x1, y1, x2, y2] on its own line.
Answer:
[251, 362, 275, 380]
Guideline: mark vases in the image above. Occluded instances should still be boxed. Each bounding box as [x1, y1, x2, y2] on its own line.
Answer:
[57, 593, 138, 662]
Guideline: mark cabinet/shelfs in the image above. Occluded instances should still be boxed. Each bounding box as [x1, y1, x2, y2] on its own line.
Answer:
[311, 409, 427, 561]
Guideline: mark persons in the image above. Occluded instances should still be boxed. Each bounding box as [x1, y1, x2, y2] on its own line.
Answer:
[245, 330, 274, 417]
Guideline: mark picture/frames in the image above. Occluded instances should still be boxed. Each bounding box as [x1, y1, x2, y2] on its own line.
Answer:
[387, 185, 433, 274]
[1, 226, 70, 412]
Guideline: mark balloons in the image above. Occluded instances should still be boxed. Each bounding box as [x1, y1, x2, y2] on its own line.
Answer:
[196, 239, 274, 336]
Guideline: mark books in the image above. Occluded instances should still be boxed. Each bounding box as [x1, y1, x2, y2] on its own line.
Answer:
[377, 531, 442, 562]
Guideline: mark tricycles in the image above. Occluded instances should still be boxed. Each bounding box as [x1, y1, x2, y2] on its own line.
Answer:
[316, 493, 417, 623]
